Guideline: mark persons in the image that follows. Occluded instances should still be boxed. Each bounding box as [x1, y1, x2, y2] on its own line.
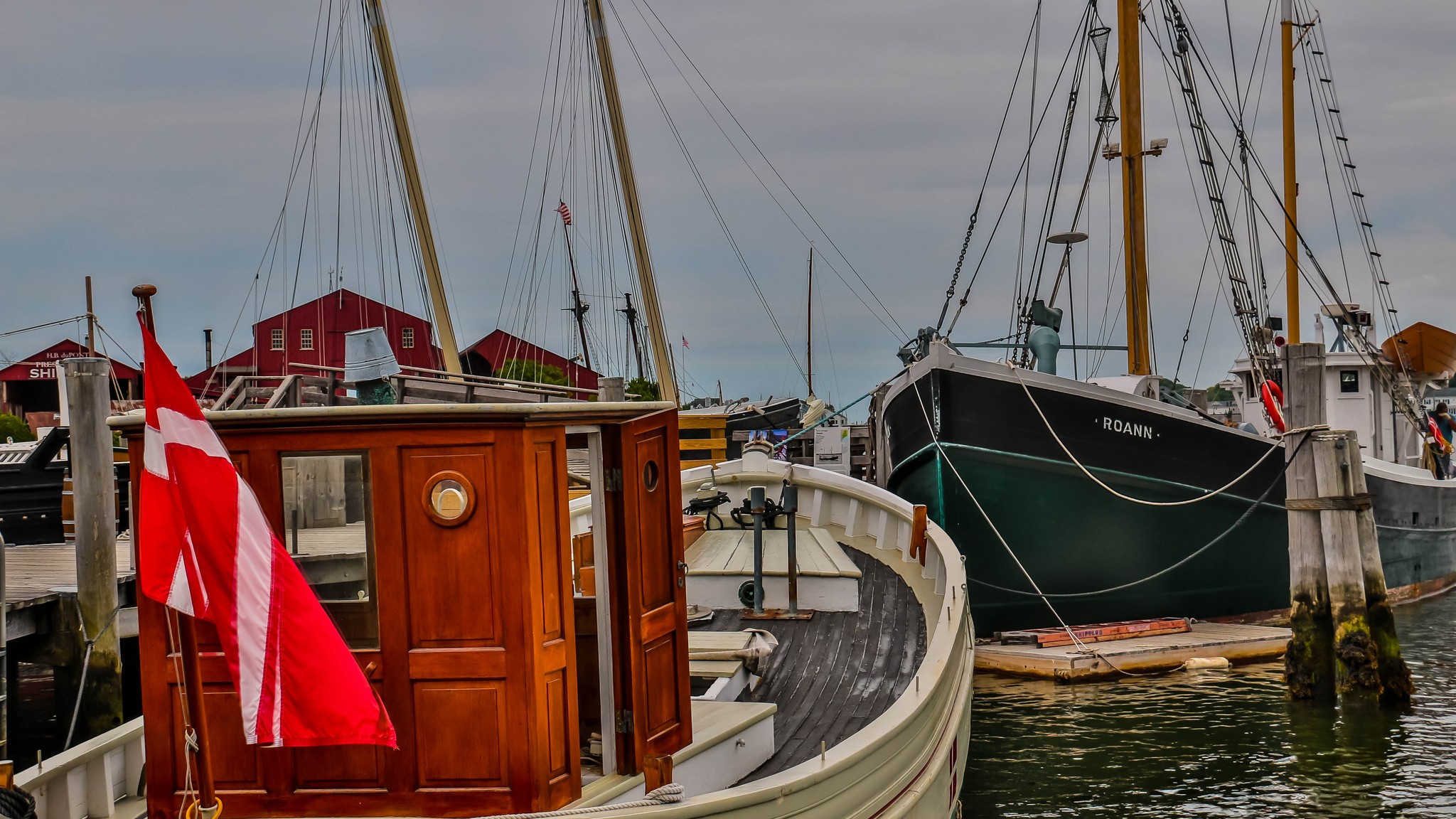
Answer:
[1421, 402, 1456, 480]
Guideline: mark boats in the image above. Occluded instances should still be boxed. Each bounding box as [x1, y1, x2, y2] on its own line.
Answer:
[885, 1, 1456, 691]
[0, 0, 979, 819]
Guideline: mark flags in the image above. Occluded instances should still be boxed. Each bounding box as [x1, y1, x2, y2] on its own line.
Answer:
[132, 310, 402, 756]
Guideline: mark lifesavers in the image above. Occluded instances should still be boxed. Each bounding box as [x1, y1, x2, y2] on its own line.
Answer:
[1261, 380, 1285, 433]
[1428, 417, 1445, 451]
